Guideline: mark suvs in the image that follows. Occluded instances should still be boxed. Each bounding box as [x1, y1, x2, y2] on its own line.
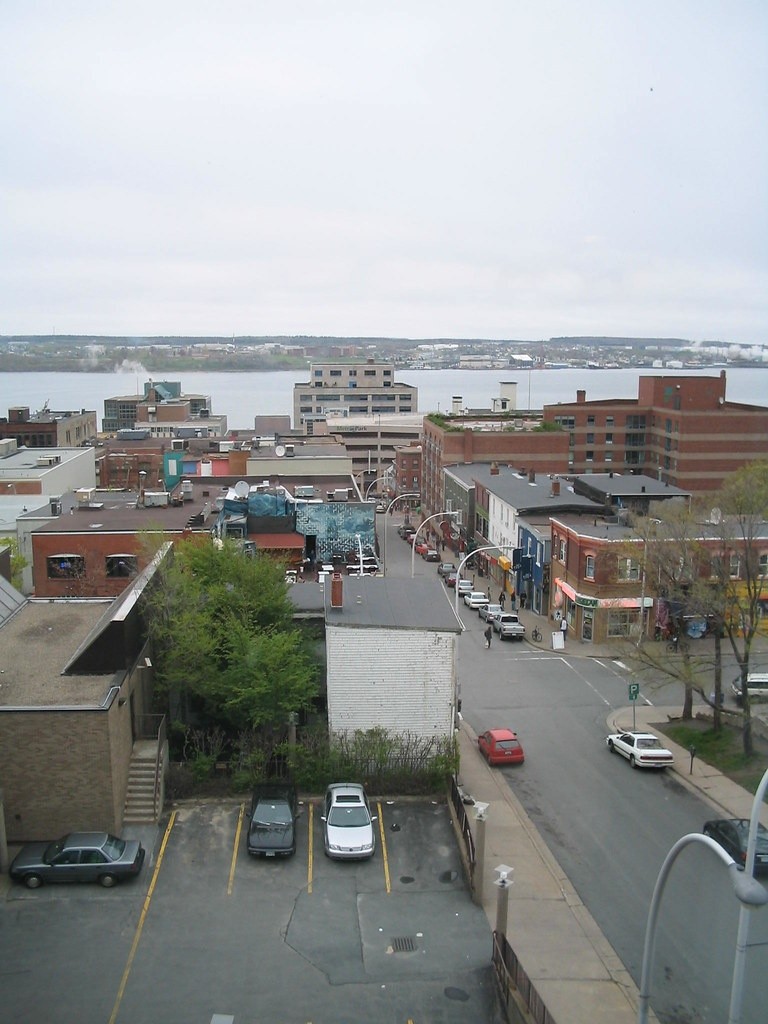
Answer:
[730, 672, 768, 705]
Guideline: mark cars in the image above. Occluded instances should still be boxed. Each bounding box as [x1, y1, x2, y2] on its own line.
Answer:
[437, 562, 458, 578]
[444, 572, 463, 588]
[605, 730, 674, 770]
[8, 830, 145, 888]
[478, 728, 525, 768]
[421, 549, 441, 562]
[320, 782, 378, 861]
[454, 580, 476, 597]
[397, 524, 433, 555]
[464, 591, 490, 610]
[245, 793, 296, 859]
[367, 497, 387, 513]
[702, 818, 768, 876]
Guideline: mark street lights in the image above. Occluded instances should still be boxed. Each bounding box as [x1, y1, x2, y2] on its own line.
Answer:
[455, 545, 518, 613]
[639, 518, 663, 631]
[411, 512, 458, 577]
[383, 494, 420, 575]
[365, 477, 392, 501]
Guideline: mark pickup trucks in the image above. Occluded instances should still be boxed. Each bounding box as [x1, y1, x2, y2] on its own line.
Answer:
[478, 604, 503, 624]
[492, 612, 528, 641]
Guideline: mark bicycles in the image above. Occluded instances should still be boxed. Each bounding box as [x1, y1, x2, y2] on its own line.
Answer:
[531, 625, 544, 642]
[666, 638, 690, 653]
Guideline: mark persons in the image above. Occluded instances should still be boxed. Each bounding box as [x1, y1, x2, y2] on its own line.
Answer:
[426, 528, 430, 541]
[560, 616, 568, 641]
[441, 537, 446, 551]
[484, 626, 492, 648]
[436, 537, 440, 552]
[511, 591, 516, 611]
[390, 505, 393, 516]
[498, 591, 506, 604]
[520, 590, 527, 608]
